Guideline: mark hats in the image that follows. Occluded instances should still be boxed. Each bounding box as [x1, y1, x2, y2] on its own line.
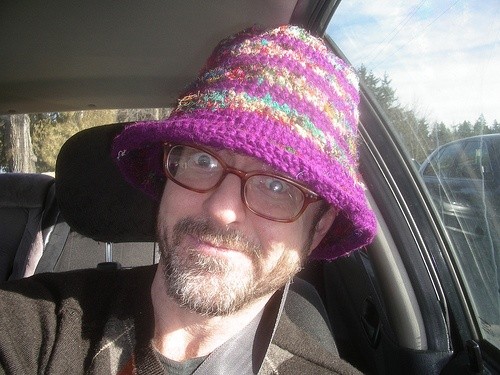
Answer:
[110, 25, 376, 260]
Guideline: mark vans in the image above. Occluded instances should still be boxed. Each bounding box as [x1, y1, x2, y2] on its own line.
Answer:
[411, 133, 499, 347]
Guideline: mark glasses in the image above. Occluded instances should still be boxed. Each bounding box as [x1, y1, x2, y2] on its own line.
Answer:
[164, 145, 324, 223]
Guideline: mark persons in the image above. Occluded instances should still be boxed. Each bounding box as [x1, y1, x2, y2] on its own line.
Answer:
[0, 23, 378, 375]
